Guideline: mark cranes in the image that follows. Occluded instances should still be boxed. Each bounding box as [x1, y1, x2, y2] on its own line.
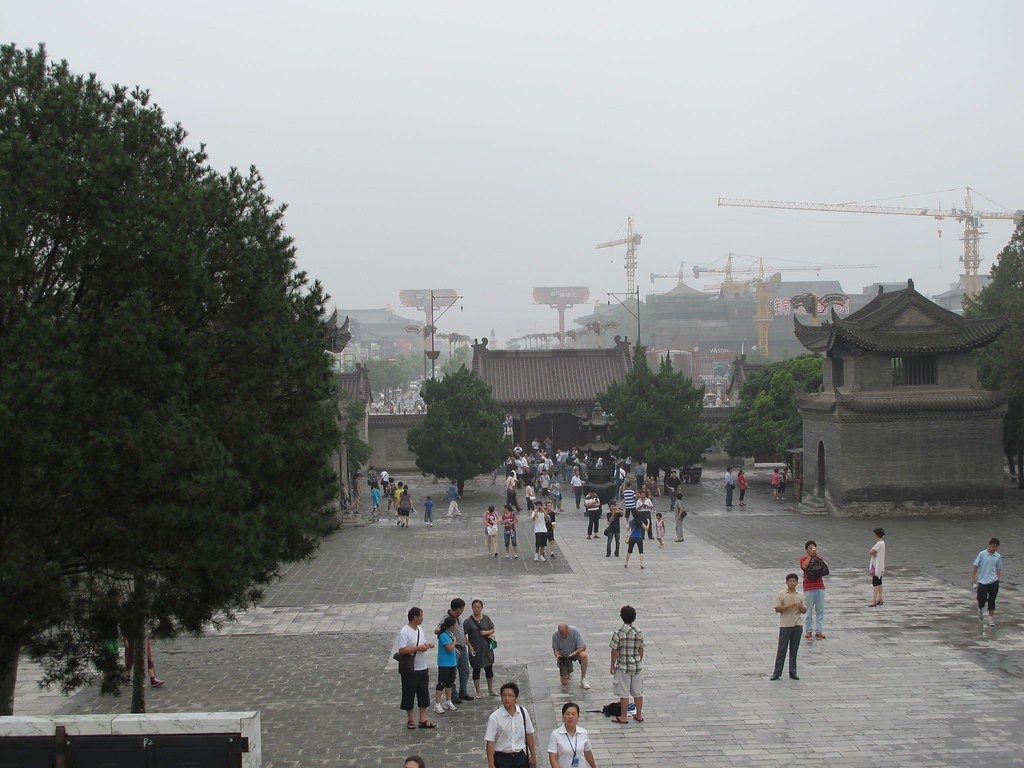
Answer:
[650, 261, 725, 287]
[692, 252, 878, 359]
[594, 216, 643, 347]
[718, 186, 1024, 302]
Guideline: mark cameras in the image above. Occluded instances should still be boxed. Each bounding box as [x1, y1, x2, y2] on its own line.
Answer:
[812, 547, 816, 551]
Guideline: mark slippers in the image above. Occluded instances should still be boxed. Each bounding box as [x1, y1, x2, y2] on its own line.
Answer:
[612, 716, 629, 723]
[634, 715, 644, 721]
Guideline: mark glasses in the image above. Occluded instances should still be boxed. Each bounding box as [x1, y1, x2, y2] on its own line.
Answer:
[502, 694, 515, 698]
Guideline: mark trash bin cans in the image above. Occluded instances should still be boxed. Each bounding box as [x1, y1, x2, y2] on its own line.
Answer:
[679, 467, 690, 483]
[690, 467, 702, 483]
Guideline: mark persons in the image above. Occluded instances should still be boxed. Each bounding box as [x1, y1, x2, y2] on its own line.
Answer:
[336, 466, 418, 529]
[424, 496, 433, 527]
[532, 501, 550, 562]
[867, 527, 885, 607]
[672, 494, 685, 542]
[500, 504, 519, 559]
[972, 538, 1003, 627]
[482, 435, 788, 569]
[371, 597, 663, 768]
[486, 683, 537, 768]
[483, 506, 500, 557]
[549, 623, 591, 689]
[547, 703, 597, 768]
[446, 479, 462, 517]
[609, 605, 644, 723]
[656, 513, 665, 548]
[800, 540, 826, 639]
[584, 488, 602, 539]
[768, 574, 800, 681]
[370, 398, 428, 412]
[544, 502, 556, 557]
[605, 500, 624, 557]
[624, 512, 650, 569]
[399, 607, 437, 730]
[94, 588, 164, 697]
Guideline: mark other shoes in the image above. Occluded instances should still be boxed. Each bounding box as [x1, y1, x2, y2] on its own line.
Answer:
[770, 674, 779, 680]
[489, 551, 556, 562]
[386, 509, 462, 527]
[815, 632, 825, 638]
[408, 690, 499, 729]
[805, 632, 812, 638]
[869, 600, 884, 606]
[586, 535, 685, 558]
[790, 672, 799, 679]
[978, 608, 984, 619]
[987, 617, 995, 626]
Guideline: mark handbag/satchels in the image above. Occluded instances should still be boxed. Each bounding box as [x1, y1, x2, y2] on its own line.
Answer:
[367, 477, 371, 484]
[380, 479, 384, 485]
[487, 524, 498, 535]
[399, 655, 415, 672]
[808, 557, 829, 577]
[681, 511, 687, 519]
[625, 536, 630, 543]
[485, 635, 497, 650]
[508, 488, 517, 497]
[604, 527, 610, 536]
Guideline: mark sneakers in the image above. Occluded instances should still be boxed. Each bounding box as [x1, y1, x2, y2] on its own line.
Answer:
[580, 677, 590, 689]
